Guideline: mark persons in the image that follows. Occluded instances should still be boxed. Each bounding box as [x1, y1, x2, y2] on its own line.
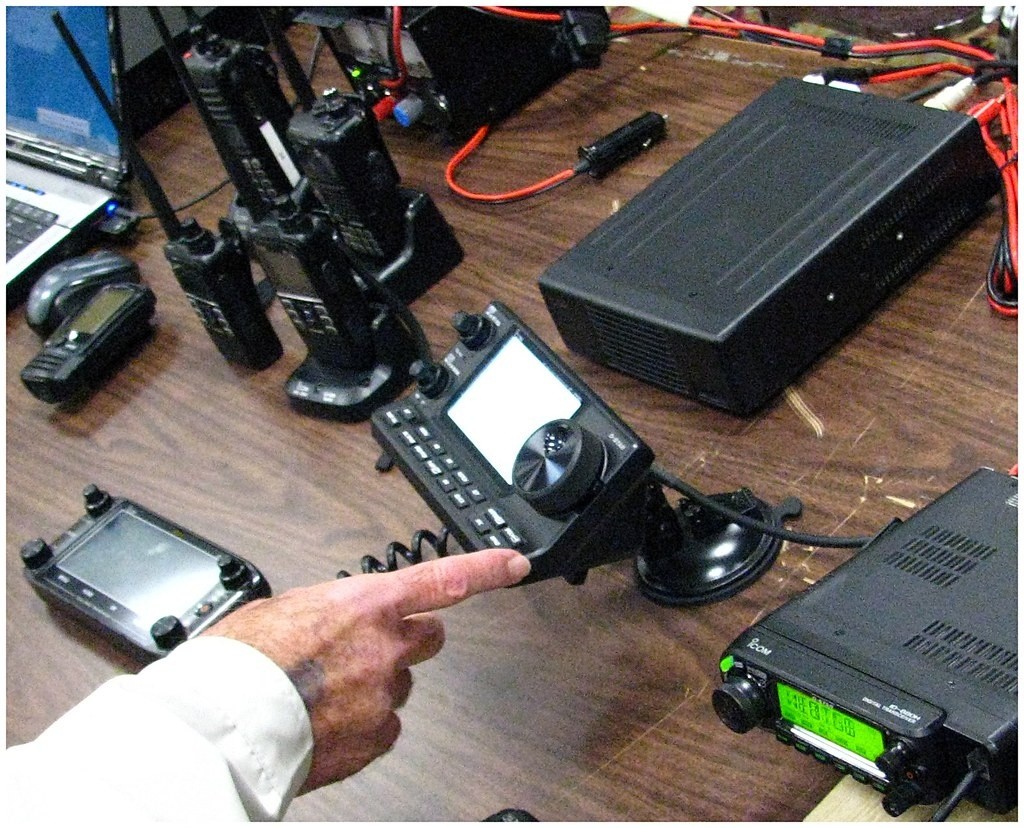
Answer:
[0, 546, 532, 828]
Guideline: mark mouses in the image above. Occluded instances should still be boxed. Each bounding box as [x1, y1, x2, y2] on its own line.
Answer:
[25, 248, 140, 338]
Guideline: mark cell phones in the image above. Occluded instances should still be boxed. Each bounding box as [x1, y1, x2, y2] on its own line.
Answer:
[19, 281, 158, 404]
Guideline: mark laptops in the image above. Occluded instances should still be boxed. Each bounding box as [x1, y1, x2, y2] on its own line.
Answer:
[6, 6, 133, 308]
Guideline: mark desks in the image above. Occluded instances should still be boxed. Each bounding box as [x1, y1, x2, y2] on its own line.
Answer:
[6, 29, 1018, 822]
